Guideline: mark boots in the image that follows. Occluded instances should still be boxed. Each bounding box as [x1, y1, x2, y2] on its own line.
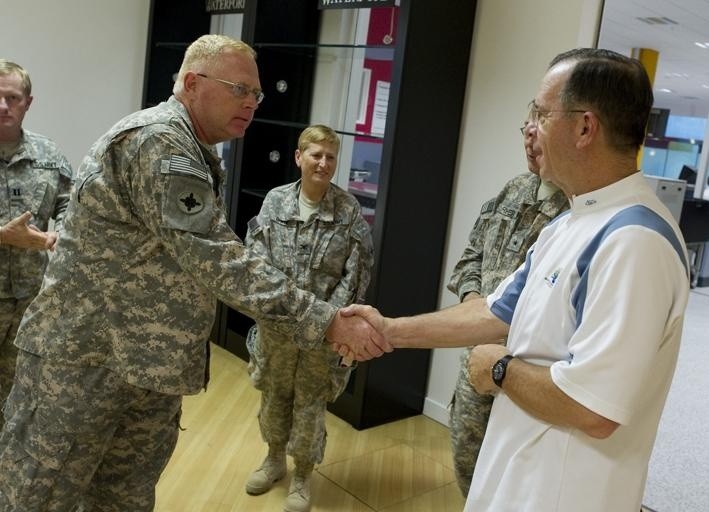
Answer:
[240, 447, 288, 496]
[282, 462, 314, 512]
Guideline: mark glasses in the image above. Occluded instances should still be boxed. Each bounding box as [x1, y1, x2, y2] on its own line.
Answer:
[525, 102, 608, 131]
[195, 72, 266, 106]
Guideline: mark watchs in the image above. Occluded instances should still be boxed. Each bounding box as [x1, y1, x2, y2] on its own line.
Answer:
[492, 353, 515, 389]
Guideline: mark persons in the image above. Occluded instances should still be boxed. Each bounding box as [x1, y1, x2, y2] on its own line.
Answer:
[446, 106, 571, 500]
[1, 60, 73, 432]
[334, 50, 691, 512]
[0, 35, 393, 511]
[243, 124, 377, 510]
